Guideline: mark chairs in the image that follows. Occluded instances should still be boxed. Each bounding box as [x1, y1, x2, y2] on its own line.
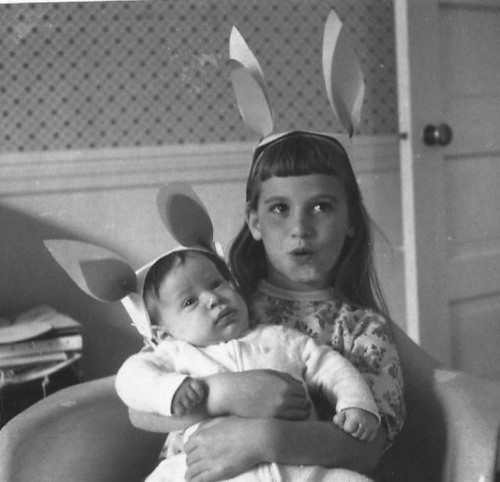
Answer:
[0, 370, 500, 481]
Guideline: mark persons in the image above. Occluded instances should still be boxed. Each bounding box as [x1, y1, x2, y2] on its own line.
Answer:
[127, 128, 408, 482]
[112, 244, 383, 482]
